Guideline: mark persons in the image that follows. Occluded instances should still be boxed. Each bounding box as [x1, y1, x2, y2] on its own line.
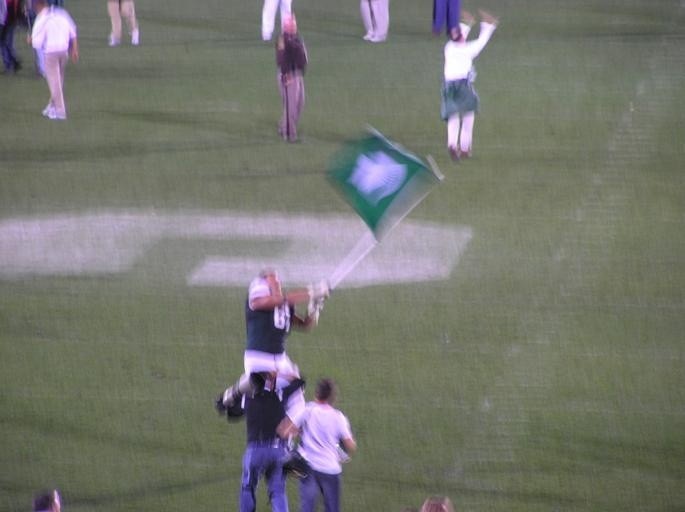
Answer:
[214, 269, 330, 420]
[261, 1, 292, 42]
[24, 0, 63, 79]
[358, 0, 391, 44]
[105, 1, 140, 48]
[275, 18, 309, 143]
[33, 489, 61, 512]
[276, 377, 356, 511]
[441, 7, 500, 162]
[433, 0, 459, 39]
[27, 0, 79, 121]
[227, 370, 296, 511]
[418, 495, 453, 512]
[0, 0, 29, 75]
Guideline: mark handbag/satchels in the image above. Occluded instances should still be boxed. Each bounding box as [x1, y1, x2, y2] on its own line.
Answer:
[285, 452, 310, 478]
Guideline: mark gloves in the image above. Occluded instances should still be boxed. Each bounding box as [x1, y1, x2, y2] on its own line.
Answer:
[308, 285, 328, 320]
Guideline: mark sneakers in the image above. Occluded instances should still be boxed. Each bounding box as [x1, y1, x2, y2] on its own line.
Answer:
[363, 33, 386, 42]
[42, 106, 66, 120]
[216, 398, 245, 417]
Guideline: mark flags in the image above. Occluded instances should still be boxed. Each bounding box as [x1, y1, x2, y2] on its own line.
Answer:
[323, 123, 443, 245]
